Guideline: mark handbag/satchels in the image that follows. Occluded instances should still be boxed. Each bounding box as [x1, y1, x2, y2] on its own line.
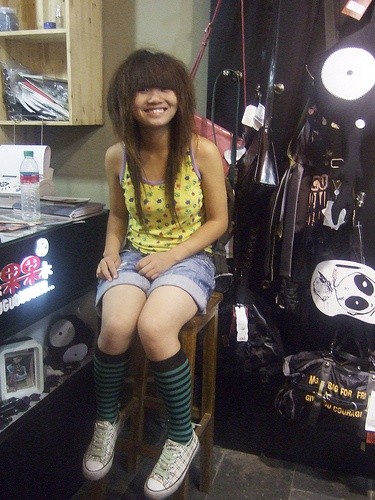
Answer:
[228, 290, 283, 369]
[276, 352, 375, 440]
[236, 127, 279, 205]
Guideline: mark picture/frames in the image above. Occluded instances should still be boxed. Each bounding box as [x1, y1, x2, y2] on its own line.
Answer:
[0, 339, 44, 401]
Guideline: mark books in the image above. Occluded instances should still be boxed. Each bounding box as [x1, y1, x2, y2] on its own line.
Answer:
[16, 72, 71, 121]
[12, 195, 108, 218]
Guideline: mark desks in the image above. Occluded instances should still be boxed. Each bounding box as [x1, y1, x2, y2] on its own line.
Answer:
[0, 196, 110, 500]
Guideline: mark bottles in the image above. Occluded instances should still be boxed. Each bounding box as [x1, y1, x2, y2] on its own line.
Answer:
[18, 150, 41, 222]
[55, 4, 63, 28]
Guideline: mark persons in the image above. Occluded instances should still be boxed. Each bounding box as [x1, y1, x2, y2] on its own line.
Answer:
[82, 47, 229, 500]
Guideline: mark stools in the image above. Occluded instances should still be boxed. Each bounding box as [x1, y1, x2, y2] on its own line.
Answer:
[91, 292, 224, 500]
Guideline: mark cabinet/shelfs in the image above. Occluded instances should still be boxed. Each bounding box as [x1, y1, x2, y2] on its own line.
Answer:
[0, 0, 102, 125]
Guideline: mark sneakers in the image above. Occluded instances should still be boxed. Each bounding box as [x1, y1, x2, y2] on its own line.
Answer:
[82, 411, 122, 482]
[144, 424, 200, 500]
